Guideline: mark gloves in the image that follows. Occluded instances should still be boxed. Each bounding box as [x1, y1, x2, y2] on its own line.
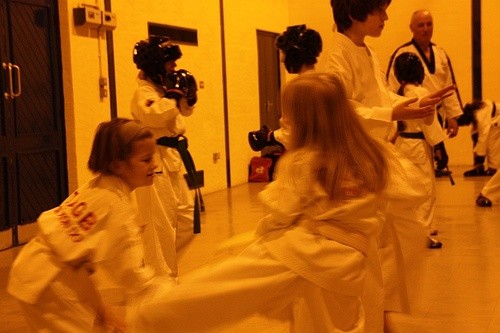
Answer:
[178, 68, 198, 107]
[164, 71, 184, 101]
[248, 124, 275, 152]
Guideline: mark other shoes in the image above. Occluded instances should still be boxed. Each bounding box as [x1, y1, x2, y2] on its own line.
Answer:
[425, 237, 442, 249]
[475, 192, 492, 207]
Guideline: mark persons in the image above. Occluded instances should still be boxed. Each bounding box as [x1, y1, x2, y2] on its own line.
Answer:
[7, 118, 179, 333]
[386, 10, 464, 144]
[460, 100, 500, 208]
[130, 35, 201, 250]
[113, 72, 435, 333]
[393, 52, 446, 249]
[322, 0, 456, 142]
[248, 24, 323, 152]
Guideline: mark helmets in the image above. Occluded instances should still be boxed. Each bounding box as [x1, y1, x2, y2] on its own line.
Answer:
[275, 24, 323, 74]
[394, 52, 425, 84]
[132, 35, 183, 84]
